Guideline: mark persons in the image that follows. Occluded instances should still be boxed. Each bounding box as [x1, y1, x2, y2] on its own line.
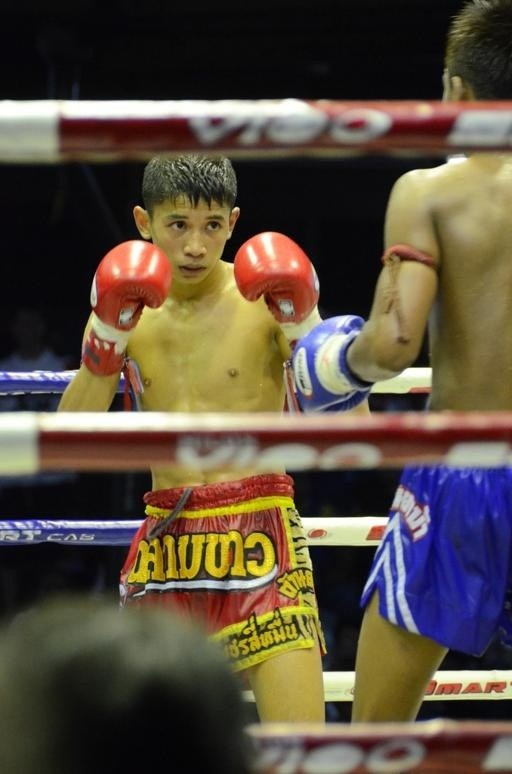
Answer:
[290, 0, 512, 725]
[0, 594, 254, 773]
[57, 152, 323, 722]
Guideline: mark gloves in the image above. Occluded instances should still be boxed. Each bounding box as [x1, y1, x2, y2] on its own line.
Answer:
[77, 237, 173, 374]
[286, 315, 379, 414]
[232, 229, 325, 326]
